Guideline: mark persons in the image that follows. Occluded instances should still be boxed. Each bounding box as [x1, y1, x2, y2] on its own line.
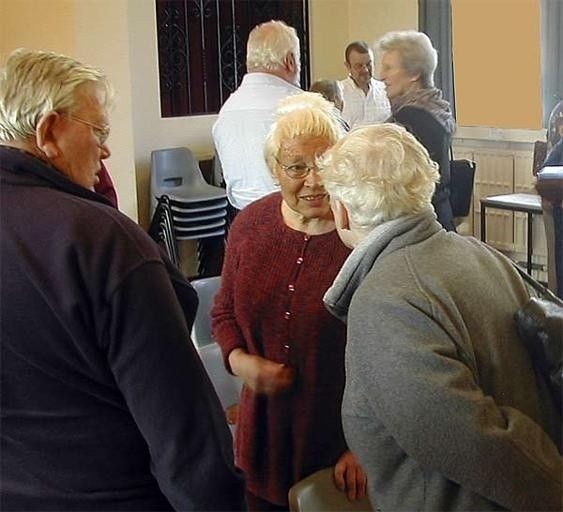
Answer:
[378, 29, 456, 234]
[0, 48, 246, 512]
[212, 21, 350, 212]
[308, 77, 352, 135]
[338, 42, 394, 128]
[316, 123, 563, 512]
[209, 91, 367, 512]
[93, 161, 116, 209]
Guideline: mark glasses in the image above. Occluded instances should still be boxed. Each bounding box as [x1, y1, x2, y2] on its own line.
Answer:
[57, 108, 111, 145]
[270, 153, 321, 181]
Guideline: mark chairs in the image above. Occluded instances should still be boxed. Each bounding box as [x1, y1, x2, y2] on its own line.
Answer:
[196, 341, 249, 436]
[137, 145, 231, 283]
[184, 274, 225, 347]
[284, 461, 371, 511]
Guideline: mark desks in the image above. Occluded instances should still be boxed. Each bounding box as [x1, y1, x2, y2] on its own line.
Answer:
[476, 192, 550, 287]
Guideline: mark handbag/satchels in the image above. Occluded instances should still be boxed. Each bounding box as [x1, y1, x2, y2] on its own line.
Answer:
[447, 144, 479, 219]
